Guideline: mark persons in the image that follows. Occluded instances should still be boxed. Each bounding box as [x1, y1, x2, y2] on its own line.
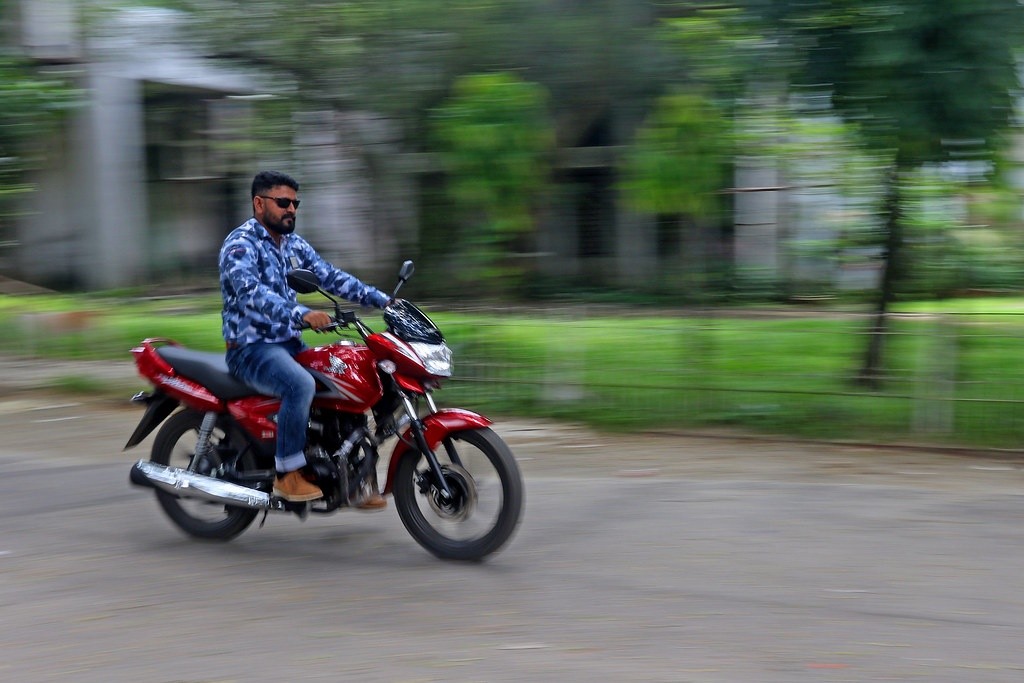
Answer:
[219, 169, 389, 511]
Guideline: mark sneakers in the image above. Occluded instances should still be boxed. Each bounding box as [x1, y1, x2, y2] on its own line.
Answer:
[273, 471, 324, 502]
[358, 498, 386, 509]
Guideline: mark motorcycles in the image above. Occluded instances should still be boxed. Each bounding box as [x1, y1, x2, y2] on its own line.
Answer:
[129, 258, 527, 563]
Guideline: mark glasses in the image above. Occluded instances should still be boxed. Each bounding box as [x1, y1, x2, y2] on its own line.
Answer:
[261, 196, 300, 210]
